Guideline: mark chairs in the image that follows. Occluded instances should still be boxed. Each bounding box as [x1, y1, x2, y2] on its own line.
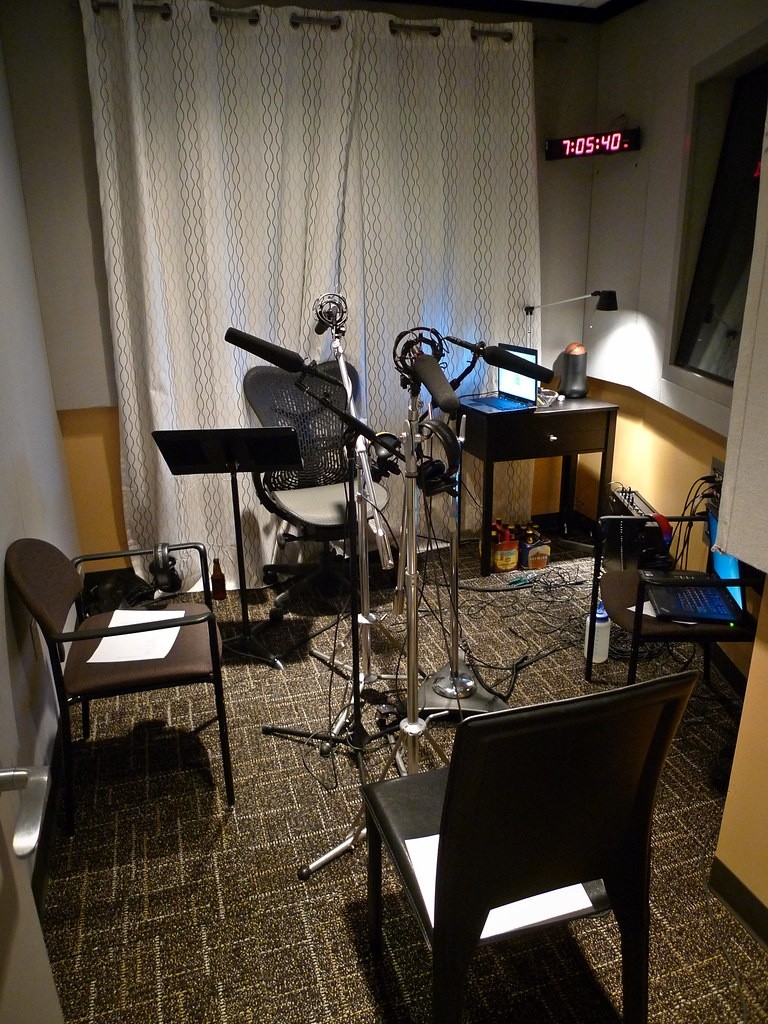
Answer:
[360, 670, 700, 1023]
[242, 359, 390, 625]
[584, 511, 765, 686]
[6, 539, 237, 808]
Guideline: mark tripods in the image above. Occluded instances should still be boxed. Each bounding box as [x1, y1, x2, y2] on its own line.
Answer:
[260, 332, 513, 881]
[149, 425, 303, 672]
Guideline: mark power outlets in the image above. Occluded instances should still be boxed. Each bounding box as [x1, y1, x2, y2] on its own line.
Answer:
[30, 616, 43, 663]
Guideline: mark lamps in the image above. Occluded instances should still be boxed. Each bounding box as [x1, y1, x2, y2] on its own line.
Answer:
[524, 289, 620, 405]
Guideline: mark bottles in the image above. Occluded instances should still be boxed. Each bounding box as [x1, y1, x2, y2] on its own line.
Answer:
[211, 558, 226, 600]
[585, 601, 610, 663]
[491, 517, 552, 572]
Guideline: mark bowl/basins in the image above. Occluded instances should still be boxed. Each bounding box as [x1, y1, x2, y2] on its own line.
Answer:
[537, 389, 558, 408]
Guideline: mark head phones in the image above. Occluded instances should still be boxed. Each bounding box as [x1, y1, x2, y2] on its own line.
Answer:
[147, 542, 182, 593]
[366, 432, 402, 478]
[416, 419, 460, 495]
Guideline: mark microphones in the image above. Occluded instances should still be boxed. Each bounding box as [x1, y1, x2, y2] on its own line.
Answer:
[408, 339, 460, 414]
[225, 326, 340, 387]
[444, 336, 555, 383]
[314, 312, 331, 334]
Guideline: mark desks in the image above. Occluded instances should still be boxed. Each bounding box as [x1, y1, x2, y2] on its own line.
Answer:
[455, 397, 620, 579]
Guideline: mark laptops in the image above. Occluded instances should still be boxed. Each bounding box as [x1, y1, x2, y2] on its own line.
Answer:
[456, 341, 538, 414]
[638, 503, 751, 625]
